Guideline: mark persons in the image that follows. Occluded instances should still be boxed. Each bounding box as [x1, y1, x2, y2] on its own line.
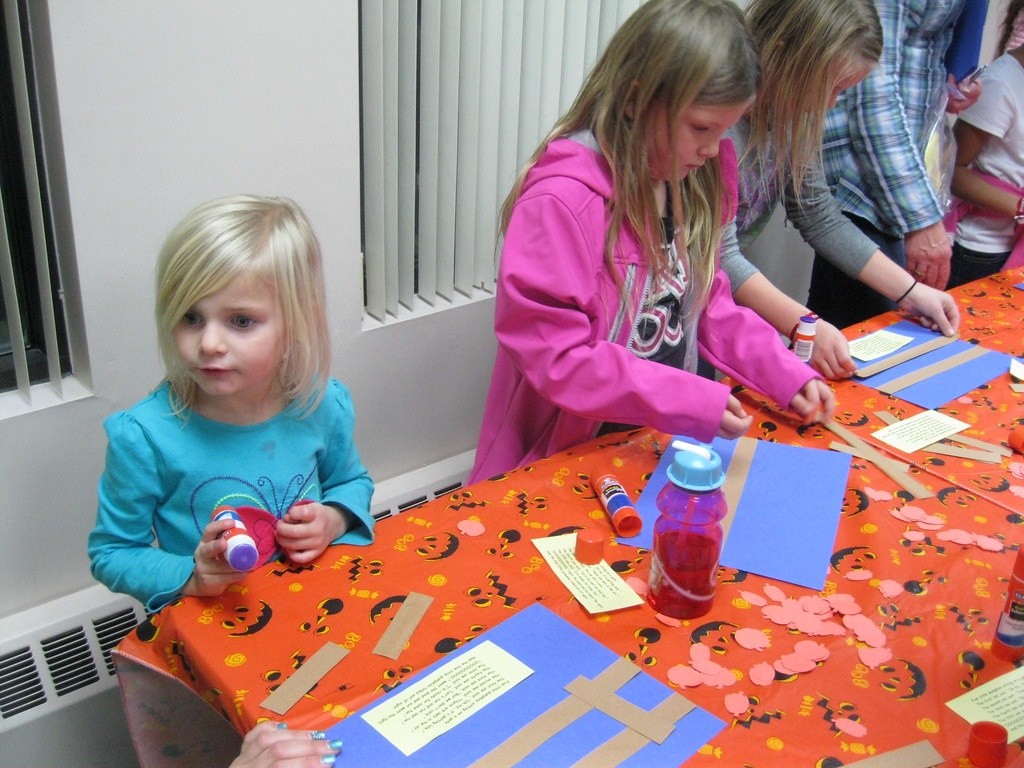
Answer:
[945, 0, 1024, 291]
[697, 0, 962, 380]
[804, 0, 983, 326]
[86, 193, 376, 613]
[226, 718, 344, 768]
[463, 0, 835, 487]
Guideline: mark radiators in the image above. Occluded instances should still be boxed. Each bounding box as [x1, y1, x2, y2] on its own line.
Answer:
[0, 449, 480, 767]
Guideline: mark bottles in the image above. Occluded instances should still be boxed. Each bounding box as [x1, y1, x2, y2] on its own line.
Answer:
[645, 439, 728, 620]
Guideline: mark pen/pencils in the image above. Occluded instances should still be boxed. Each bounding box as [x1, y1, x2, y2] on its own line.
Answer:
[971, 63, 989, 83]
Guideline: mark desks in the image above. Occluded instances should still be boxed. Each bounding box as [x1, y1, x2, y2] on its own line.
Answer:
[112, 267, 1024, 767]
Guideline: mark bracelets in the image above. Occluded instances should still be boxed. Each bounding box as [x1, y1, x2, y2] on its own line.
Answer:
[894, 279, 918, 310]
[789, 310, 821, 344]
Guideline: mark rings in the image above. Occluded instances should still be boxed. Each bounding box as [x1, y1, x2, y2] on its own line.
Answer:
[916, 270, 927, 277]
